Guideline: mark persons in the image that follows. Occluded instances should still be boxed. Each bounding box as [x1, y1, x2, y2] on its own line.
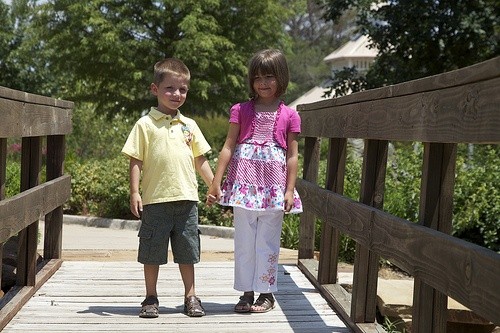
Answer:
[206, 49, 302, 313]
[119, 58, 223, 317]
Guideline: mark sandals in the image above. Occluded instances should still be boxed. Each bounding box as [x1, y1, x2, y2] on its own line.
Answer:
[139, 296, 159, 317]
[184, 296, 206, 316]
[233, 293, 277, 312]
